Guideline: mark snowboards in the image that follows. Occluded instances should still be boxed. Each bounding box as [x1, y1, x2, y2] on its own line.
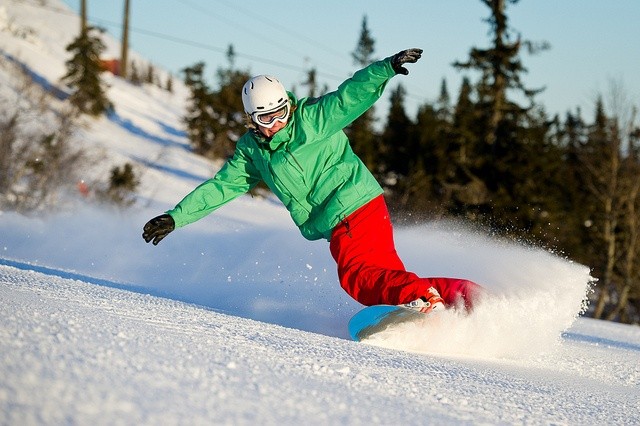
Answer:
[348, 304, 426, 342]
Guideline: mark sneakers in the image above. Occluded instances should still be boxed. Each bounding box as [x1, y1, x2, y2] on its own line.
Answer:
[396, 286, 446, 313]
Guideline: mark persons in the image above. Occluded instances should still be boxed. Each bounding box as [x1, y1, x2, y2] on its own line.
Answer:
[142, 49, 489, 317]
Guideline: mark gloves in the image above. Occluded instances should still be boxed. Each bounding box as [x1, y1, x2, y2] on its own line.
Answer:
[391, 47, 423, 75]
[143, 214, 174, 245]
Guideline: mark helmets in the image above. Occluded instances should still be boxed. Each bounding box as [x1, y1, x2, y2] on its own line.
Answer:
[242, 74, 292, 118]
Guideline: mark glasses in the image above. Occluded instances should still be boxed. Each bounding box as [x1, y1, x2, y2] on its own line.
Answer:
[244, 99, 291, 128]
